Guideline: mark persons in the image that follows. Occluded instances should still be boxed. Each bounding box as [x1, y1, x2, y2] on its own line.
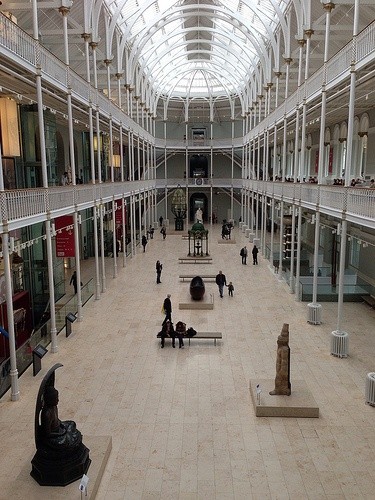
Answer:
[226, 282, 234, 297]
[146, 224, 155, 240]
[159, 216, 163, 227]
[216, 270, 226, 298]
[246, 174, 375, 188]
[69, 271, 83, 295]
[176, 321, 186, 350]
[61, 172, 83, 186]
[252, 245, 259, 265]
[160, 224, 167, 240]
[238, 216, 244, 222]
[240, 246, 248, 265]
[160, 321, 176, 349]
[221, 222, 234, 239]
[270, 324, 292, 396]
[142, 235, 147, 252]
[41, 387, 82, 457]
[156, 260, 163, 284]
[209, 212, 218, 224]
[161, 293, 174, 326]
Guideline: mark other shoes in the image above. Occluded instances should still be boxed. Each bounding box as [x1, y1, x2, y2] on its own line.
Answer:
[221, 295, 223, 298]
[172, 343, 176, 348]
[161, 343, 164, 348]
[159, 282, 161, 283]
[157, 282, 158, 284]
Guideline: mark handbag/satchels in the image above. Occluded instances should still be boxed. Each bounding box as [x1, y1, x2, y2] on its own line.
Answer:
[161, 304, 166, 314]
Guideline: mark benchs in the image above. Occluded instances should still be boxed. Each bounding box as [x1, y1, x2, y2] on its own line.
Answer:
[157, 332, 222, 346]
[179, 274, 216, 282]
[178, 258, 212, 263]
